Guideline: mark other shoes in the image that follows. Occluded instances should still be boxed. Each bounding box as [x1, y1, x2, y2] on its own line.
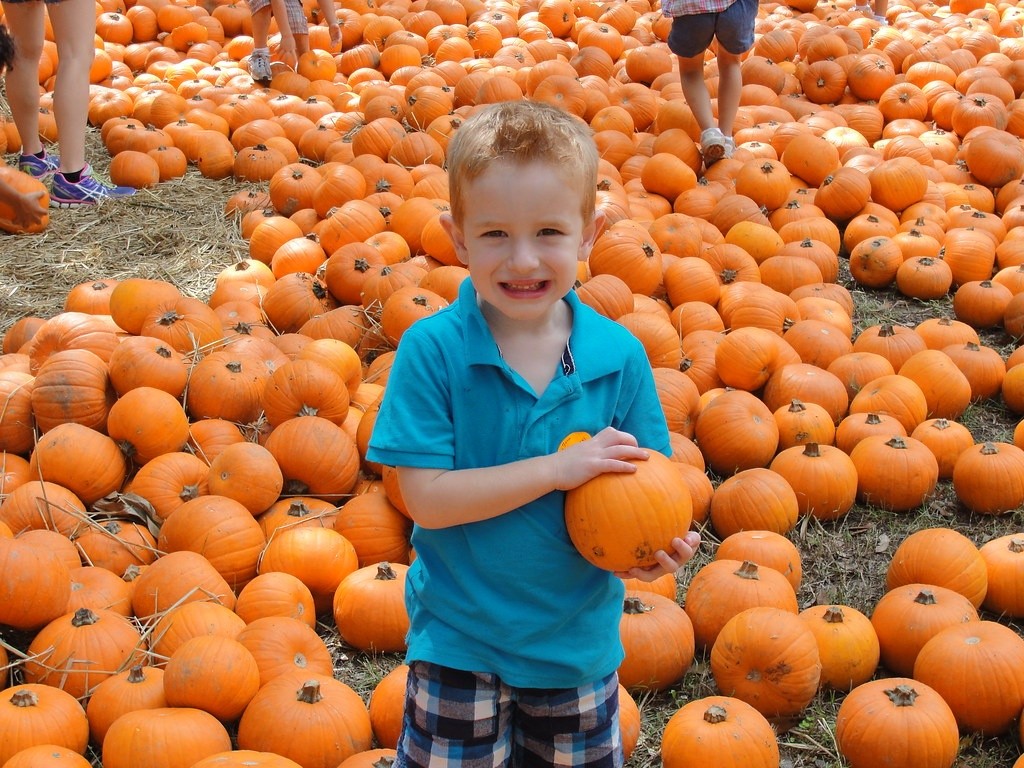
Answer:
[723, 136, 735, 159]
[701, 128, 724, 168]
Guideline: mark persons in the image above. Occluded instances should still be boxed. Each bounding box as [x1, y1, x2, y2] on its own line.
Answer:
[243, 0, 342, 82]
[660, 0, 759, 169]
[364, 102, 701, 768]
[851, 0, 889, 25]
[0, 0, 137, 208]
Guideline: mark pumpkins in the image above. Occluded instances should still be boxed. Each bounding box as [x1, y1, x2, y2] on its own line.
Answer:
[0, 0, 1023, 768]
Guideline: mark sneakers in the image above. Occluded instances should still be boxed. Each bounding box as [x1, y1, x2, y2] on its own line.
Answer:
[248, 49, 272, 82]
[50, 163, 136, 208]
[20, 144, 60, 182]
